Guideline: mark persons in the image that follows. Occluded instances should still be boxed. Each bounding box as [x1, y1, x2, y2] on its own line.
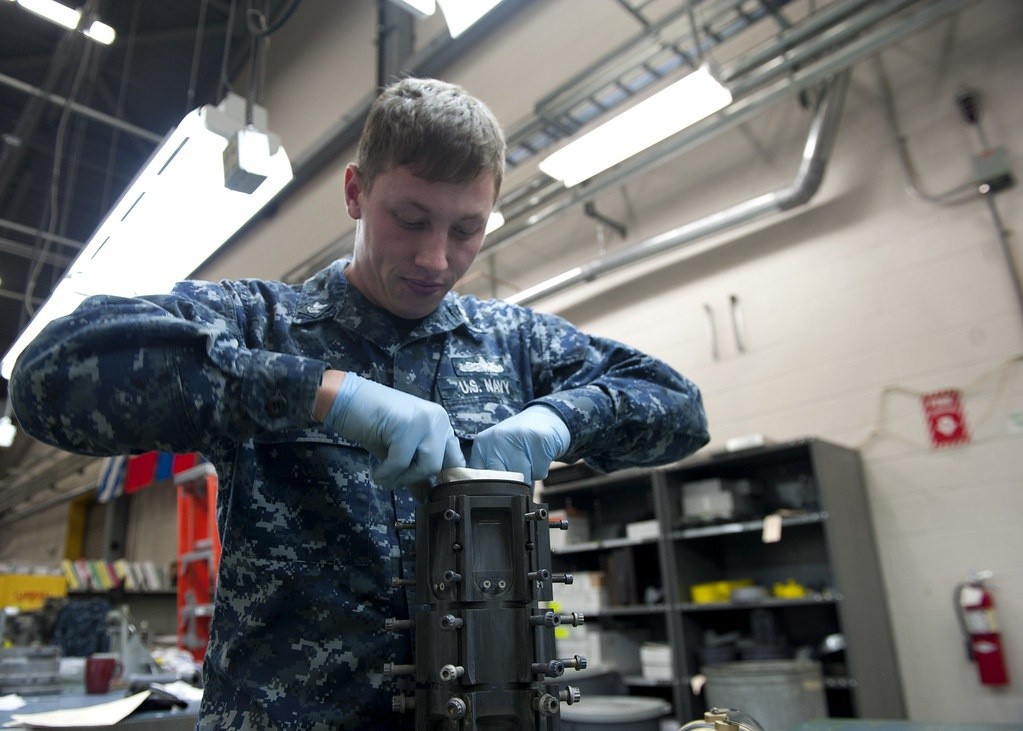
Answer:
[9, 77, 712, 731]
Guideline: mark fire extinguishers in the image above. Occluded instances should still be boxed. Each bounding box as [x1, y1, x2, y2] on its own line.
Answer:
[952, 574, 1010, 685]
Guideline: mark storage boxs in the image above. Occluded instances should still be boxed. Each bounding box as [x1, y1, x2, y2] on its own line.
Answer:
[555, 624, 675, 679]
[548, 508, 662, 549]
[691, 579, 755, 603]
[683, 480, 750, 523]
[554, 550, 632, 611]
[557, 697, 674, 731]
[700, 659, 826, 721]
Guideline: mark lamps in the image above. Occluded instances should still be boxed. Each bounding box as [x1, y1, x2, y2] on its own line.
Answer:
[538, 63, 736, 187]
[1, 93, 294, 384]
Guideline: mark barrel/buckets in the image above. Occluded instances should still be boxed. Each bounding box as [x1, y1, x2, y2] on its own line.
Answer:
[704, 661, 828, 731]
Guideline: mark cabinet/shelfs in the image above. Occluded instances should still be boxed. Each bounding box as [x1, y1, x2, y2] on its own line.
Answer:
[661, 436, 909, 723]
[531, 467, 684, 721]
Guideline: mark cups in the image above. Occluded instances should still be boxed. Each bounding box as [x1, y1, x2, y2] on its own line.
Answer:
[85, 657, 123, 695]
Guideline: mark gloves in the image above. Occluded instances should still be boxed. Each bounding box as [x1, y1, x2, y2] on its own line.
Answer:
[470, 404, 571, 487]
[323, 372, 466, 488]
